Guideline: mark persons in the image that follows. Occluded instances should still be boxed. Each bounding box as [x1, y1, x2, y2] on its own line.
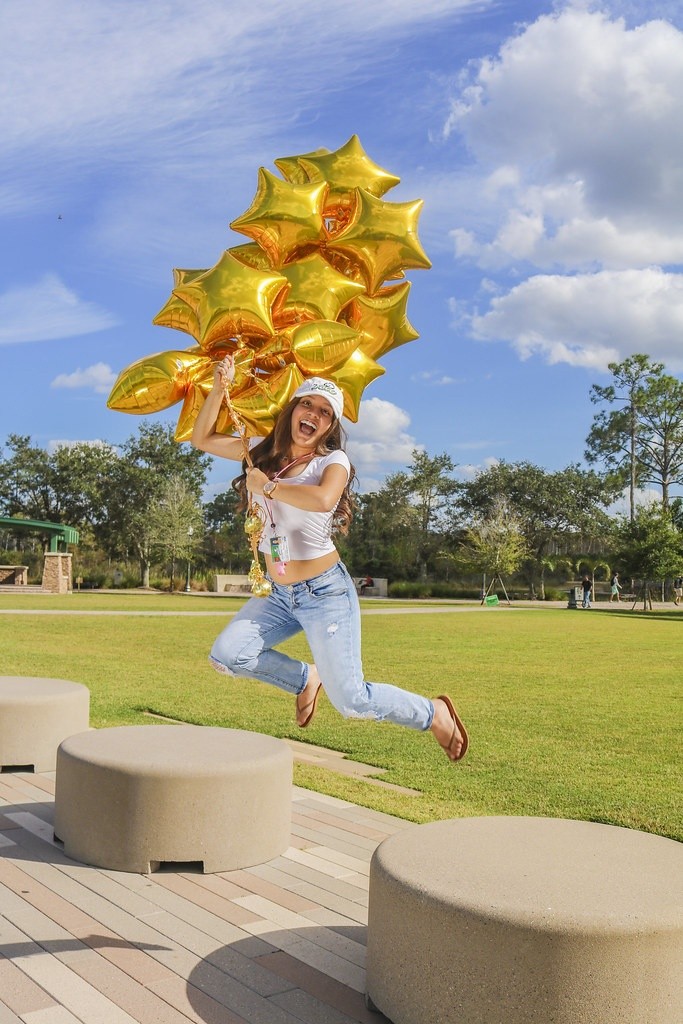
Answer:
[581, 575, 593, 608]
[672, 575, 683, 606]
[357, 576, 374, 595]
[609, 573, 622, 603]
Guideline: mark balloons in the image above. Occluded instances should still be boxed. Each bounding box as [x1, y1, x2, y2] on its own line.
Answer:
[109, 134, 434, 439]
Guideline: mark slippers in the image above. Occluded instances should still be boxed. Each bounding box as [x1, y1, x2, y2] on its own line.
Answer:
[435, 695, 469, 761]
[294, 681, 325, 728]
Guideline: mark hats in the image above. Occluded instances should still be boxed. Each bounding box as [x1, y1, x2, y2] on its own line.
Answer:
[289, 377, 344, 422]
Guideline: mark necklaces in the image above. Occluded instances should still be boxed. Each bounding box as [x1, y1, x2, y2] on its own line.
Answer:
[191, 357, 469, 762]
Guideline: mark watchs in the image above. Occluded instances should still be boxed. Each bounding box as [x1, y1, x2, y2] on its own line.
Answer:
[262, 480, 278, 499]
